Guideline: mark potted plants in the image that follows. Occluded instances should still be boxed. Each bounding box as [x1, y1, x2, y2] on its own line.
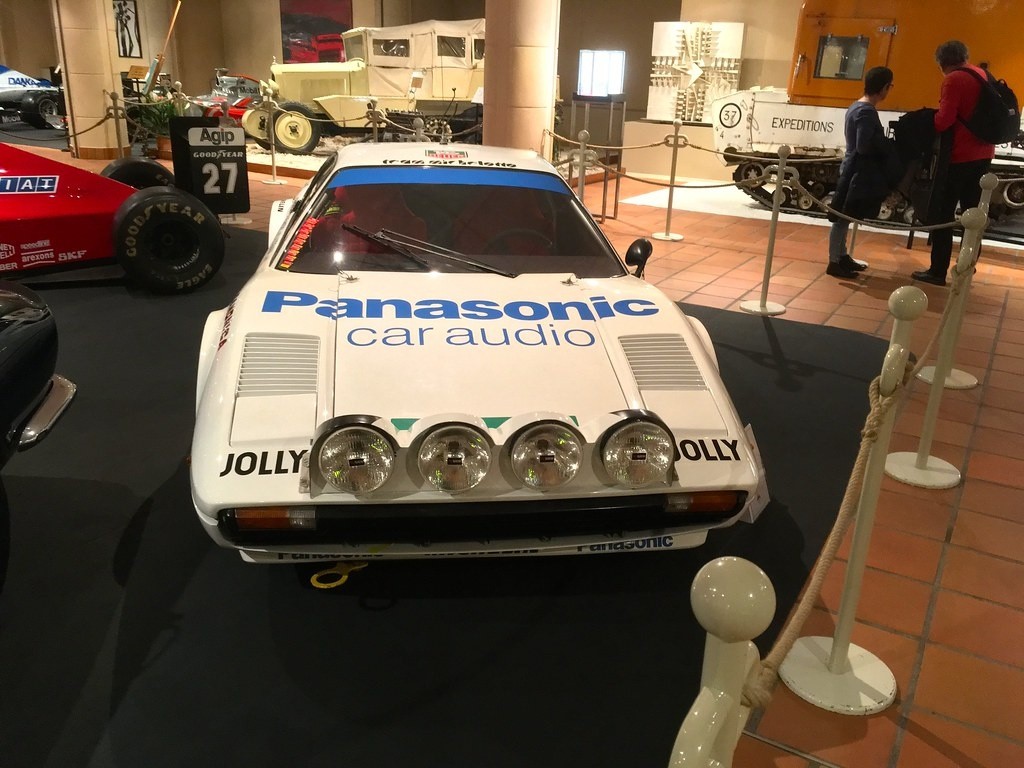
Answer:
[141, 98, 179, 160]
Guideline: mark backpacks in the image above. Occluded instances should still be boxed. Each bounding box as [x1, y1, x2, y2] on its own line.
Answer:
[951, 65, 1020, 144]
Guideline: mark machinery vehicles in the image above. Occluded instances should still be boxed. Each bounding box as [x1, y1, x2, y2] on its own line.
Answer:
[709, 0, 1024, 238]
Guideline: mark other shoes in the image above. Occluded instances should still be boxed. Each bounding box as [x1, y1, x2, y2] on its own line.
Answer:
[826, 261, 860, 279]
[840, 254, 868, 271]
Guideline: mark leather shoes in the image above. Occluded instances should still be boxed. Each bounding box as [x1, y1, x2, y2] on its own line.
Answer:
[911, 270, 946, 286]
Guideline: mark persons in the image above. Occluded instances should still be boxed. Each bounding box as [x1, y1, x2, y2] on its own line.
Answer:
[826, 66, 893, 279]
[912, 41, 995, 286]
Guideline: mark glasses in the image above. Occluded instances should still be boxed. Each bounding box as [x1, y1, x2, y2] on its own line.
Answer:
[888, 83, 894, 88]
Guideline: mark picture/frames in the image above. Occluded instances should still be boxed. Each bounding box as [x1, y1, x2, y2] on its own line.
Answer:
[112, 0, 143, 58]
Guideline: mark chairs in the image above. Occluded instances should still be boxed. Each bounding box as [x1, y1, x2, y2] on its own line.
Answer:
[452, 188, 555, 256]
[310, 184, 427, 254]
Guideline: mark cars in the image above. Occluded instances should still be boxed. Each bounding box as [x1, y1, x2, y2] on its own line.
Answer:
[189, 141, 769, 570]
[0, 63, 65, 129]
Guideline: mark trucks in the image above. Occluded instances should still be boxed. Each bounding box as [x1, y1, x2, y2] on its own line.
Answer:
[242, 15, 566, 165]
